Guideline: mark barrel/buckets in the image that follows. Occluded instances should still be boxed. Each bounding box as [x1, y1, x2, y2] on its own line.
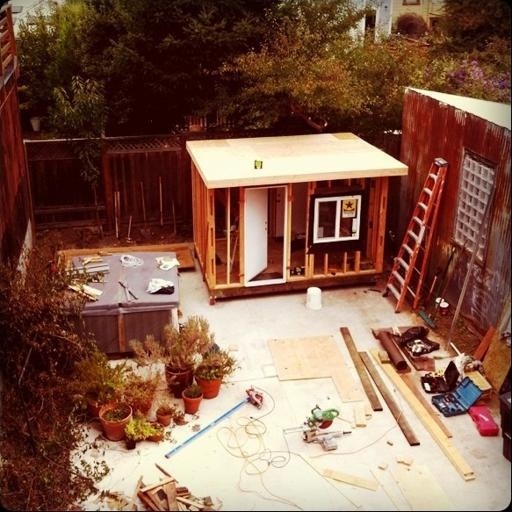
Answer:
[305, 286, 322, 310]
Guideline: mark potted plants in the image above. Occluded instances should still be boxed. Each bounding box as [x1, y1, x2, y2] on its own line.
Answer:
[84, 314, 236, 449]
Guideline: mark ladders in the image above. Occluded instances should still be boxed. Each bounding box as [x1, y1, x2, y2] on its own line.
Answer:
[382, 157, 449, 313]
